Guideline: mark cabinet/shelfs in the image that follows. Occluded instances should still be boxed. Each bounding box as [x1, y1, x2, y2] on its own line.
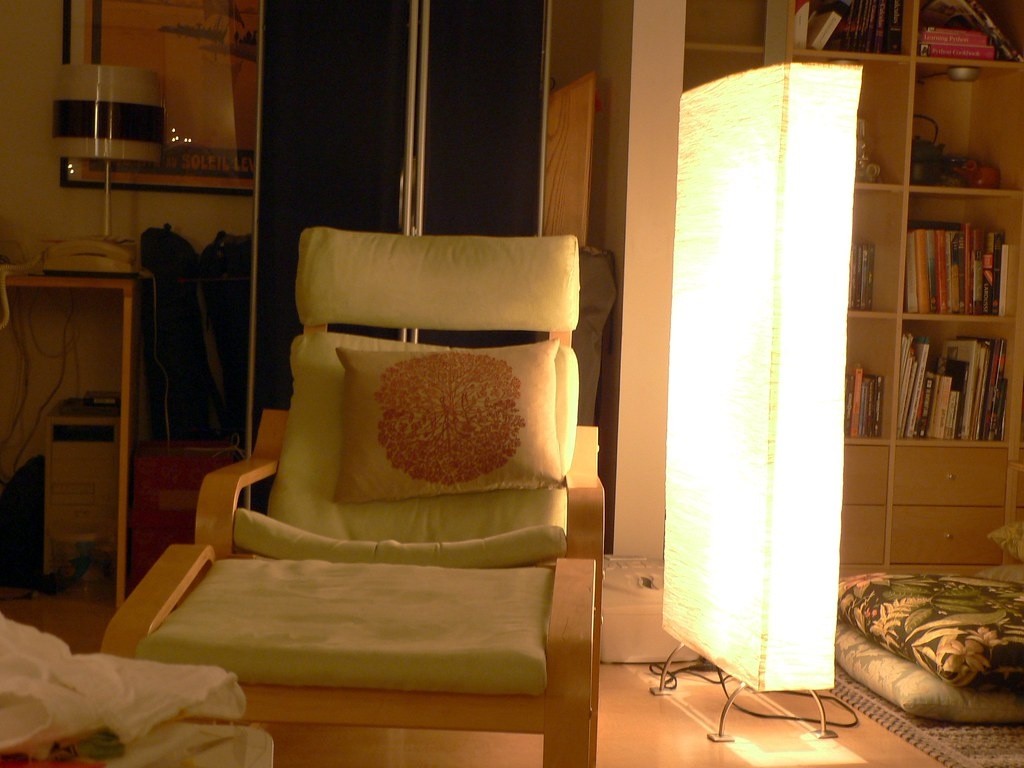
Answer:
[684, 0, 1024, 586]
[244, 0, 552, 513]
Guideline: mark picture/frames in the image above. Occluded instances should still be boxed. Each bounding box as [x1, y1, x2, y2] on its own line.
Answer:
[60, 0, 262, 195]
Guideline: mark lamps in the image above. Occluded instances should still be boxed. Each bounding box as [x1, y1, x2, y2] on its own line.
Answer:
[52, 63, 165, 237]
[652, 61, 861, 739]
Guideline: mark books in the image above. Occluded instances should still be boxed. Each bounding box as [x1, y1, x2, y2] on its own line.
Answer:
[794, 0, 904, 54]
[906, 221, 1008, 316]
[919, 0, 1024, 63]
[849, 242, 875, 310]
[845, 368, 883, 437]
[899, 332, 1007, 441]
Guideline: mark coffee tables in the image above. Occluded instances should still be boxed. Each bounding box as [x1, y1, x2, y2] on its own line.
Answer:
[0, 723, 273, 768]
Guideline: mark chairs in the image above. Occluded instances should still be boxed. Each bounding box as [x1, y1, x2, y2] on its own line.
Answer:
[98, 228, 605, 768]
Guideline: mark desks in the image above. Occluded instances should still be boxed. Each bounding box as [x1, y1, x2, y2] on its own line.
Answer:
[2, 276, 139, 608]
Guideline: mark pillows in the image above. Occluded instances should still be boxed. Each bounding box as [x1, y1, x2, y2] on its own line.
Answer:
[335, 338, 567, 505]
[835, 520, 1024, 723]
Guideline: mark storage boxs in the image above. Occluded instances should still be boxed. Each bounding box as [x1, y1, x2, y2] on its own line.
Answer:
[918, 25, 996, 59]
[129, 438, 239, 592]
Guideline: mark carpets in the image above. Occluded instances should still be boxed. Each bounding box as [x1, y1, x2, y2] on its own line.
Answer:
[831, 659, 1024, 768]
